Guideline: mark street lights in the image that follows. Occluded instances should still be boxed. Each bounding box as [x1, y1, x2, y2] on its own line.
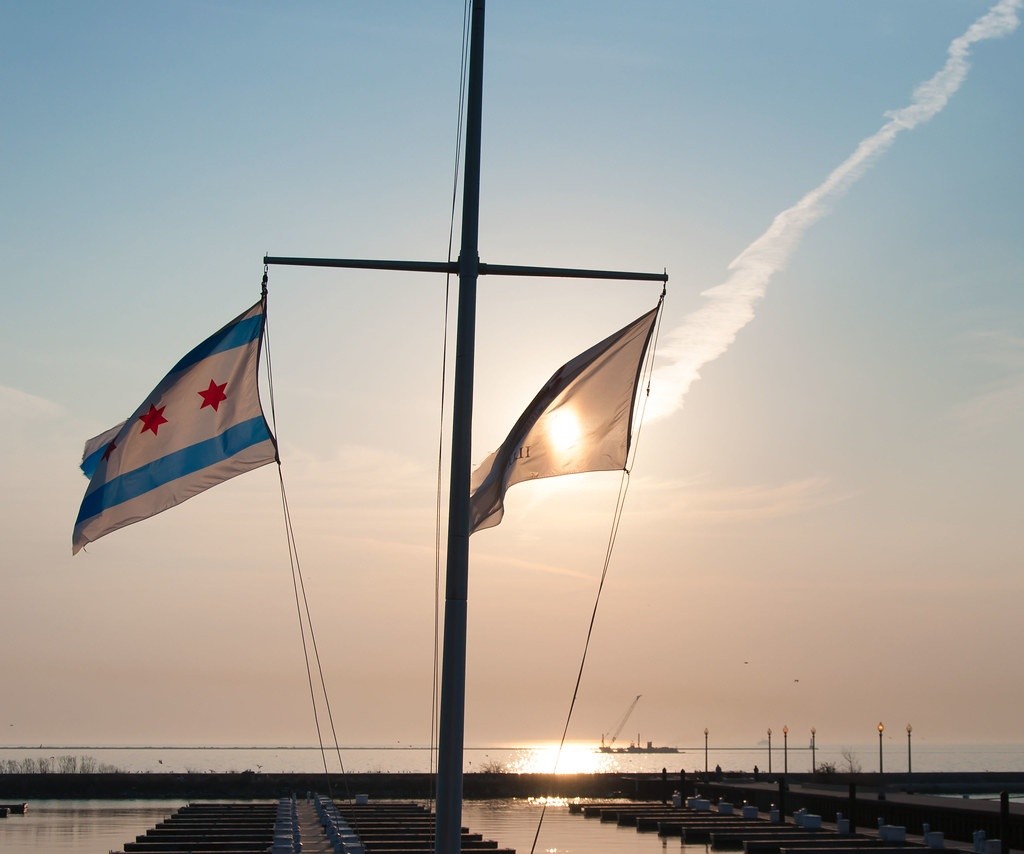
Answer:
[906, 723, 912, 773]
[767, 728, 773, 774]
[704, 729, 708, 773]
[783, 725, 789, 774]
[811, 725, 817, 773]
[878, 722, 884, 774]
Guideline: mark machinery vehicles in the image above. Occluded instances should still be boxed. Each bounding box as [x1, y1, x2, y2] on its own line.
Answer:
[599, 695, 640, 751]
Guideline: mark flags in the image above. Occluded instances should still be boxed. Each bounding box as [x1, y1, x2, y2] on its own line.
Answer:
[72, 299, 276, 556]
[469, 307, 658, 535]
